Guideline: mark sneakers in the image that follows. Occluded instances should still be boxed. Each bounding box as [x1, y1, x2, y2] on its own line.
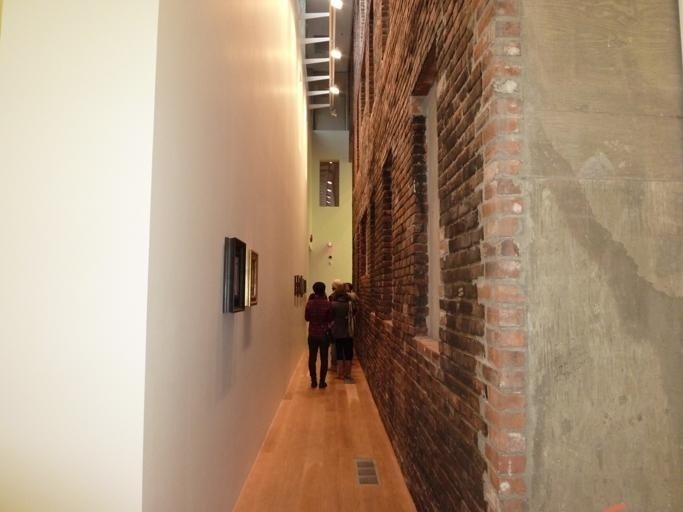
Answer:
[311, 382, 327, 387]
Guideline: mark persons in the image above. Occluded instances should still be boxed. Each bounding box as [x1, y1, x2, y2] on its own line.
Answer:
[326, 279, 357, 372]
[329, 284, 355, 381]
[304, 281, 334, 388]
[343, 281, 359, 306]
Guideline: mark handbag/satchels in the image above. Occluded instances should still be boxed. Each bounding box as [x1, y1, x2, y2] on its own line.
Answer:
[348, 312, 360, 341]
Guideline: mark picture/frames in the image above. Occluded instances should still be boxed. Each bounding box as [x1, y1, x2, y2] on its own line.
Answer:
[245, 249, 258, 307]
[224, 237, 246, 313]
